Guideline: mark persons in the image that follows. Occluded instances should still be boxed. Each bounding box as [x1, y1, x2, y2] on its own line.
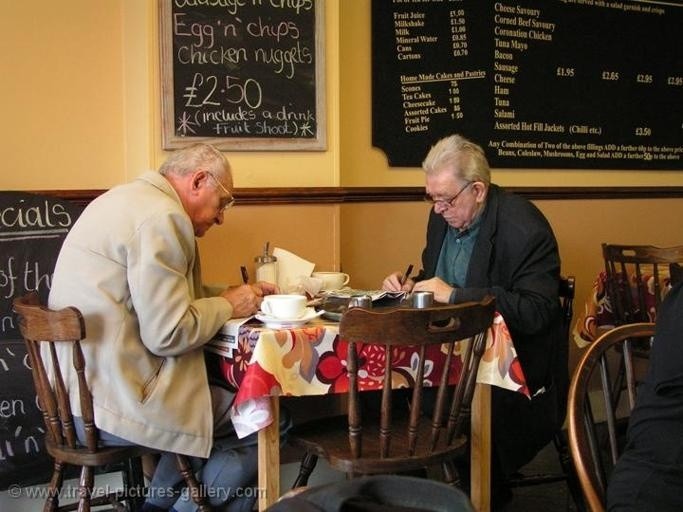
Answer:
[39, 142, 294, 512]
[606, 282, 683, 512]
[380, 134, 561, 512]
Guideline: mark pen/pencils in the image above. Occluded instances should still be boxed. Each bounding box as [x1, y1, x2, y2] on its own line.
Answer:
[400, 264, 414, 285]
[240, 265, 249, 284]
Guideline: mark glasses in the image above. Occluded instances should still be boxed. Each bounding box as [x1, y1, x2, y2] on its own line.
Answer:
[423, 177, 477, 208]
[206, 171, 235, 213]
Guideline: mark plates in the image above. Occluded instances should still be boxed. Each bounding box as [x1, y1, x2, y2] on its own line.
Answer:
[256, 314, 314, 326]
[313, 303, 347, 323]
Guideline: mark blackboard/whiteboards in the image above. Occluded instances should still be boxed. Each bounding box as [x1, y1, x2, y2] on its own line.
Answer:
[0, 190, 123, 491]
[158, 0, 328, 152]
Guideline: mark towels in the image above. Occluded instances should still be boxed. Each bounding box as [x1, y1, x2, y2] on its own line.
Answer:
[272, 246, 316, 295]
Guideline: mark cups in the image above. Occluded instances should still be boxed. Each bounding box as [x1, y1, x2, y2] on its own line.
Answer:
[261, 294, 307, 319]
[312, 270, 350, 289]
[349, 294, 373, 311]
[410, 290, 435, 309]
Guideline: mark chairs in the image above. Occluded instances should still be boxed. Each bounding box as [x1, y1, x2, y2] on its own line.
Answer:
[286, 291, 496, 494]
[564, 320, 661, 511]
[9, 288, 206, 512]
[256, 471, 477, 512]
[596, 238, 681, 467]
[496, 269, 575, 511]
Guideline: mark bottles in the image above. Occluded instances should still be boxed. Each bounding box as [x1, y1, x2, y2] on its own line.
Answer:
[254, 242, 278, 286]
